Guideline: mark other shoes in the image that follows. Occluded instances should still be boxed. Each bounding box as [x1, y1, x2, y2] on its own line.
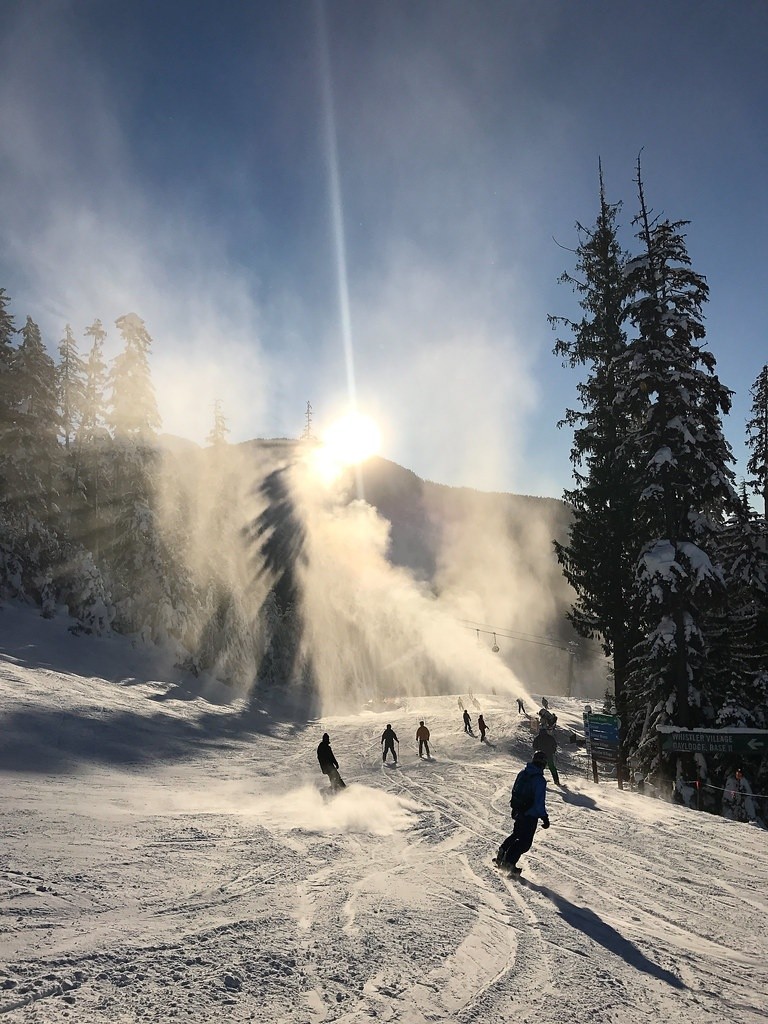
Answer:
[494, 847, 506, 867]
[502, 854, 521, 873]
[554, 780, 559, 784]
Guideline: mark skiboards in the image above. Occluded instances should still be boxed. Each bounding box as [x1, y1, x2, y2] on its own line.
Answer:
[546, 779, 568, 787]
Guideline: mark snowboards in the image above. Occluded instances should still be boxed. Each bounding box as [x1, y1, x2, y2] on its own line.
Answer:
[492, 857, 523, 877]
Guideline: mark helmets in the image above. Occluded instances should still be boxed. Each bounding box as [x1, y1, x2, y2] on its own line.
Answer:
[533, 750, 548, 765]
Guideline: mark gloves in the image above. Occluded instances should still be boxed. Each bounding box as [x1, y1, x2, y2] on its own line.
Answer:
[541, 814, 550, 829]
[335, 762, 339, 768]
[511, 808, 518, 819]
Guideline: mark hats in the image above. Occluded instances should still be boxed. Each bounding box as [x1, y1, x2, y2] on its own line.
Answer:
[539, 728, 546, 734]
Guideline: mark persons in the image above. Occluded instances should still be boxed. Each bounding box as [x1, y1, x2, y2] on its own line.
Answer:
[416, 721, 430, 757]
[381, 724, 399, 763]
[533, 729, 559, 785]
[492, 686, 497, 696]
[317, 733, 346, 790]
[463, 710, 472, 732]
[478, 715, 489, 742]
[491, 751, 550, 878]
[541, 697, 552, 709]
[468, 685, 474, 696]
[516, 696, 526, 713]
[472, 698, 480, 710]
[458, 696, 465, 712]
